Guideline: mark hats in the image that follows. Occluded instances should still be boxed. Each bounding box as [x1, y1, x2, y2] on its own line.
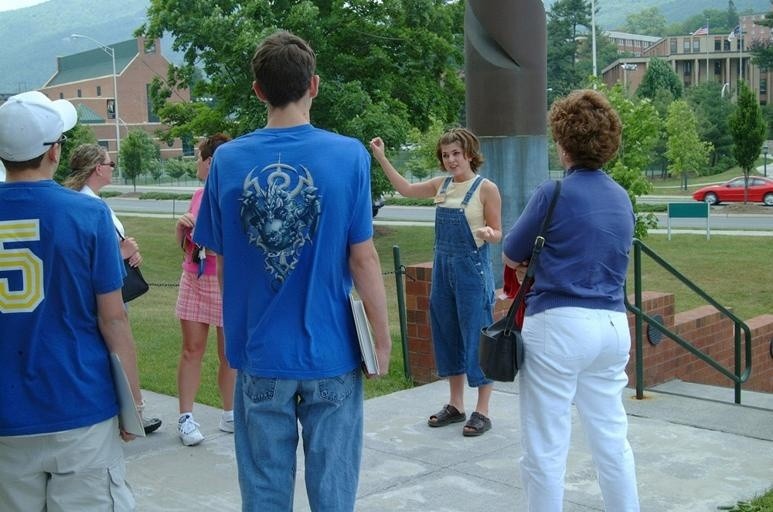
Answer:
[0, 90, 77, 162]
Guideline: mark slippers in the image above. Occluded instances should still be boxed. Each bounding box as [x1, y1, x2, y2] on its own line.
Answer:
[428, 404, 466, 427]
[463, 412, 491, 436]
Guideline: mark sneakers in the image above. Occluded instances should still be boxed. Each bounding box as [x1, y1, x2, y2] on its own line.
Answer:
[143, 416, 161, 434]
[220, 411, 235, 433]
[177, 414, 204, 446]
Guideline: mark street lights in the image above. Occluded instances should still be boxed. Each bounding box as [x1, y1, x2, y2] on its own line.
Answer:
[728, 24, 742, 96]
[70, 34, 122, 180]
[762, 146, 768, 177]
[689, 33, 708, 82]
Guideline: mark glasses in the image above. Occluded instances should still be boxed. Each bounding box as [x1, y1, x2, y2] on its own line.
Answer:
[101, 160, 115, 168]
[44, 134, 67, 147]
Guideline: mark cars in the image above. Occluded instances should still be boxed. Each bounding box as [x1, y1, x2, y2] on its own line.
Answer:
[692, 176, 773, 206]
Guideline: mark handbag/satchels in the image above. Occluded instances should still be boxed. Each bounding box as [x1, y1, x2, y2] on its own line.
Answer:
[479, 317, 522, 381]
[121, 258, 149, 302]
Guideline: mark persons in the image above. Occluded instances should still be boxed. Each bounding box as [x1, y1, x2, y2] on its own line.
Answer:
[369, 127, 503, 436]
[1, 88, 146, 511]
[500, 89, 640, 511]
[189, 30, 392, 511]
[174, 133, 234, 447]
[61, 142, 163, 436]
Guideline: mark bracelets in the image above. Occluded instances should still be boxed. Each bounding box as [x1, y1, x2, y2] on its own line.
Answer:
[136, 400, 147, 413]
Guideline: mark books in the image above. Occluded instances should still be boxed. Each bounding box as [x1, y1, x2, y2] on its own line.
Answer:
[348, 292, 380, 377]
